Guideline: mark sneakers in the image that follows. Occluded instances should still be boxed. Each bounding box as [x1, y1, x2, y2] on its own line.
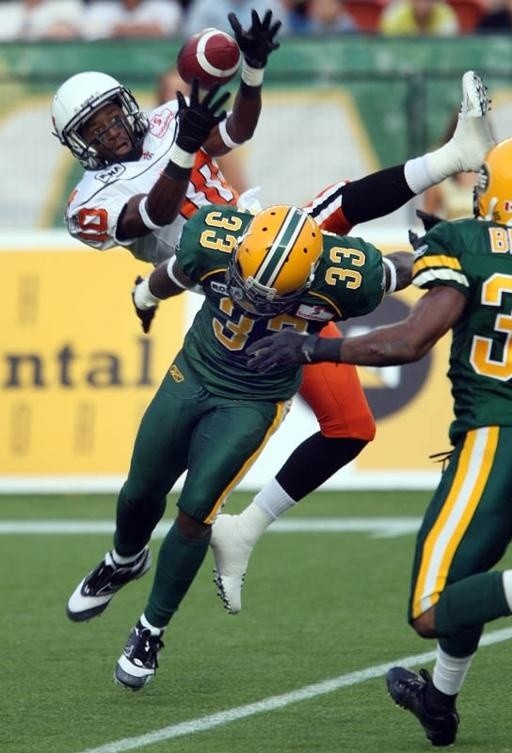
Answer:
[210, 512, 246, 616]
[385, 664, 460, 747]
[113, 610, 163, 692]
[459, 70, 502, 173]
[66, 545, 154, 624]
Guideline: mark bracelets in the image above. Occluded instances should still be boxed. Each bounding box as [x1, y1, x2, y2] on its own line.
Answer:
[169, 144, 195, 169]
[139, 196, 166, 231]
[383, 257, 399, 295]
[217, 111, 242, 150]
[135, 275, 161, 312]
[240, 59, 266, 89]
[166, 254, 185, 288]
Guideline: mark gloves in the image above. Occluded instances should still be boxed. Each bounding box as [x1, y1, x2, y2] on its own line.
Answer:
[168, 78, 231, 169]
[228, 6, 284, 97]
[244, 332, 342, 376]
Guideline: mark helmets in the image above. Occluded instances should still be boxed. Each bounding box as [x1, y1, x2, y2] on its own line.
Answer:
[473, 138, 512, 229]
[225, 204, 324, 321]
[49, 70, 150, 169]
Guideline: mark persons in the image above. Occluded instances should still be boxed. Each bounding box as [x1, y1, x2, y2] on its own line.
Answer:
[68, 202, 416, 692]
[50, 10, 492, 613]
[247, 136, 512, 745]
[0, 0, 512, 42]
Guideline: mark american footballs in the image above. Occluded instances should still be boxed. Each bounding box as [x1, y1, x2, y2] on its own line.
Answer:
[176, 28, 241, 91]
